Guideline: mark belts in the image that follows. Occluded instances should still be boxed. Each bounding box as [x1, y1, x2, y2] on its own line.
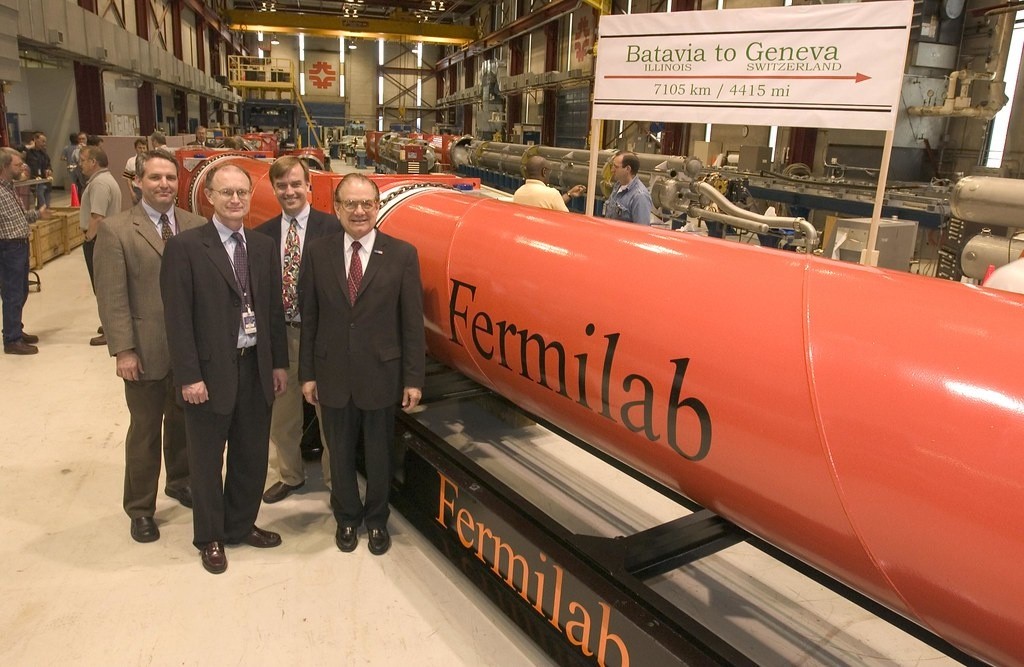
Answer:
[286, 321, 300, 328]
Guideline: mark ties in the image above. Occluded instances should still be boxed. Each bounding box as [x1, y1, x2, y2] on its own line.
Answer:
[231, 233, 253, 318]
[348, 241, 363, 305]
[282, 219, 301, 317]
[160, 214, 173, 245]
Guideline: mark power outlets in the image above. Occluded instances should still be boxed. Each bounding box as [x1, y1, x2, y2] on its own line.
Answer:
[831, 158, 837, 164]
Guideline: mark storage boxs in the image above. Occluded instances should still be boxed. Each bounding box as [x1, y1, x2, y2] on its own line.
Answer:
[27, 206, 86, 269]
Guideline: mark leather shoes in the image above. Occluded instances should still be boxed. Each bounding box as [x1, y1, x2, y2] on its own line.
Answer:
[131, 517, 160, 544]
[367, 526, 390, 555]
[165, 486, 192, 508]
[227, 524, 282, 548]
[201, 542, 228, 574]
[335, 524, 358, 552]
[263, 481, 305, 503]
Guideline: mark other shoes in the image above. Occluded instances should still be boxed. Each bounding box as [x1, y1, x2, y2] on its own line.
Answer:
[90, 334, 107, 345]
[4, 339, 38, 354]
[22, 331, 38, 343]
[97, 326, 104, 334]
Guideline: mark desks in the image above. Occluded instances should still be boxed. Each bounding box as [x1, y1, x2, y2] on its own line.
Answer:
[13, 177, 52, 293]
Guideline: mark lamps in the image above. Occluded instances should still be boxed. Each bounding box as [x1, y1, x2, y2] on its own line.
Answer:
[260, 0, 276, 11]
[430, 0, 445, 11]
[270, 32, 279, 45]
[348, 37, 357, 49]
[411, 42, 418, 53]
[344, 8, 358, 17]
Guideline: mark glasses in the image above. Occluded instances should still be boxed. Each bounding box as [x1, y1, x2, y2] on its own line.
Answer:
[335, 200, 377, 209]
[212, 189, 253, 201]
[543, 167, 552, 174]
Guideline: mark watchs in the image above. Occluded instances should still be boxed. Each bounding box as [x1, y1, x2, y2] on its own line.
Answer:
[566, 192, 572, 199]
[84, 232, 92, 242]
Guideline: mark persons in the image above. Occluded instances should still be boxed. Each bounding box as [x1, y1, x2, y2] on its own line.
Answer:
[92, 151, 208, 546]
[513, 156, 586, 213]
[14, 125, 215, 213]
[249, 156, 343, 504]
[77, 145, 121, 345]
[0, 147, 45, 355]
[298, 176, 427, 555]
[159, 164, 290, 574]
[604, 150, 652, 226]
